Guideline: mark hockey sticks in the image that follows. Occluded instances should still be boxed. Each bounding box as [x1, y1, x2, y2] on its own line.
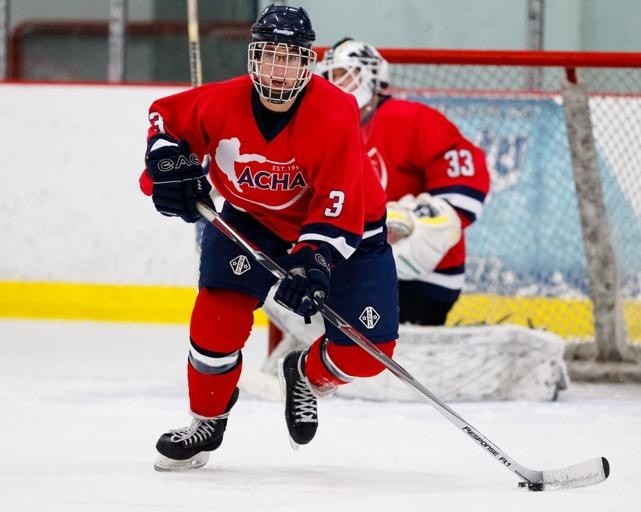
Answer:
[140, 170, 609, 491]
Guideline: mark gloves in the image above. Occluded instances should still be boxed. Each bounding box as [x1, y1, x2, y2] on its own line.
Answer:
[145, 144, 219, 224]
[273, 240, 333, 319]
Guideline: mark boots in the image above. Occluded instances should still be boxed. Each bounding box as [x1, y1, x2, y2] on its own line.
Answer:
[155, 384, 241, 462]
[283, 344, 321, 445]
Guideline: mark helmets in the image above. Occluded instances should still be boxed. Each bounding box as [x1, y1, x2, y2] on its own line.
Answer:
[251, 4, 316, 66]
[322, 37, 389, 89]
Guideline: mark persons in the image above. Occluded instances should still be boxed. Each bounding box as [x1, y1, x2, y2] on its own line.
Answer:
[319, 38, 491, 325]
[145, 4, 399, 460]
